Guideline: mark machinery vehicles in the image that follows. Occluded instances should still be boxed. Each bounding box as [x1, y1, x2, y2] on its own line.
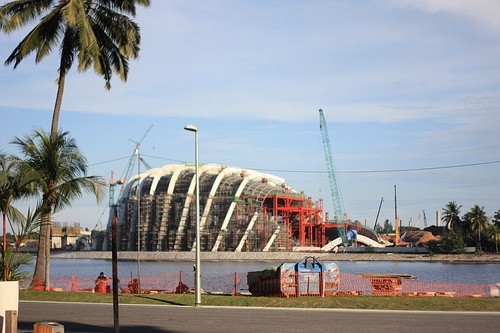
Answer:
[389, 184, 408, 247]
[316, 108, 350, 247]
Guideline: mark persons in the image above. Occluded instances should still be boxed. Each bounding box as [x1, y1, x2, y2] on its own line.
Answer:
[176, 281, 189, 294]
[94, 271, 111, 293]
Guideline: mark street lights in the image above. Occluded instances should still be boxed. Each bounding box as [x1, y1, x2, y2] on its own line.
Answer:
[184, 124, 203, 306]
